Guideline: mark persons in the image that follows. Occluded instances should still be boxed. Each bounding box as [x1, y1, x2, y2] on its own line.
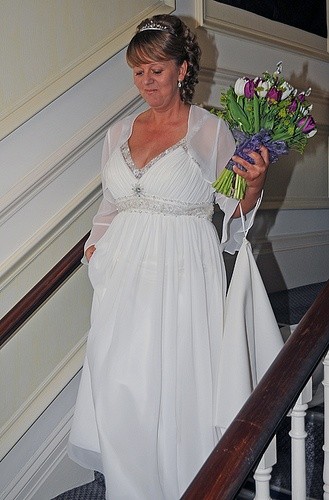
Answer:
[66, 14, 285, 500]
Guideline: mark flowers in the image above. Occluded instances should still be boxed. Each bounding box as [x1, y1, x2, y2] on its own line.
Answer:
[210, 60, 320, 199]
